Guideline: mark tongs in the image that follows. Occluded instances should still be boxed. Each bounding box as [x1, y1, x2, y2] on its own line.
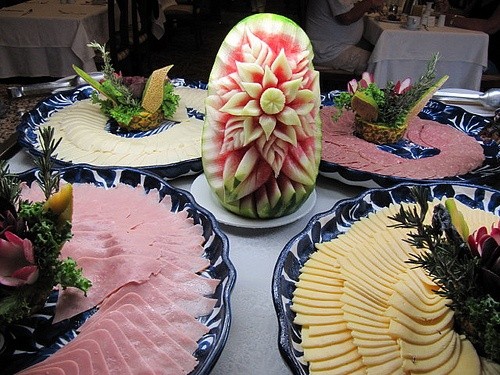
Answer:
[432, 88, 500, 111]
[8, 70, 106, 98]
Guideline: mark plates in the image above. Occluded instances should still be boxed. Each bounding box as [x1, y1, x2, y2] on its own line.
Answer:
[317, 90, 500, 188]
[403, 25, 422, 31]
[435, 88, 496, 117]
[382, 18, 407, 24]
[16, 79, 209, 180]
[272, 183, 500, 375]
[192, 173, 318, 228]
[0, 161, 237, 375]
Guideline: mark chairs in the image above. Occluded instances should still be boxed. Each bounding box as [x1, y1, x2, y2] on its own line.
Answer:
[164, 0, 204, 48]
[95, 0, 129, 75]
[118, 0, 153, 70]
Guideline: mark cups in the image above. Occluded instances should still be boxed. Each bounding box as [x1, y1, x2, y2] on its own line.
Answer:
[408, 16, 421, 27]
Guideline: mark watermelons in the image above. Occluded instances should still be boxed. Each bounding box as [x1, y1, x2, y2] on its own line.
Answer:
[201, 13, 322, 218]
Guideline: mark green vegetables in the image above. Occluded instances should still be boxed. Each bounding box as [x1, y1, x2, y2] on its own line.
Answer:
[88, 41, 181, 124]
[0, 124, 94, 323]
[330, 49, 443, 131]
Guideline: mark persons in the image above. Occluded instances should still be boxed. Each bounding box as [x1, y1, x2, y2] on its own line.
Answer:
[306, 0, 384, 73]
[436, 0, 500, 75]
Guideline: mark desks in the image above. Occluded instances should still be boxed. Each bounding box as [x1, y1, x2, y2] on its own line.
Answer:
[0, 0, 121, 79]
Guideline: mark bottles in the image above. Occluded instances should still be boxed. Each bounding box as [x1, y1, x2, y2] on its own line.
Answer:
[420, 2, 446, 28]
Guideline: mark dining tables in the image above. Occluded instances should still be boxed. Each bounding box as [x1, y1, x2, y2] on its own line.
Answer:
[363, 12, 490, 91]
[0, 82, 364, 375]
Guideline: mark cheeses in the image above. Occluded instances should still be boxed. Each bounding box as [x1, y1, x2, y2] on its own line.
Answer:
[33, 86, 209, 168]
[292, 196, 500, 375]
